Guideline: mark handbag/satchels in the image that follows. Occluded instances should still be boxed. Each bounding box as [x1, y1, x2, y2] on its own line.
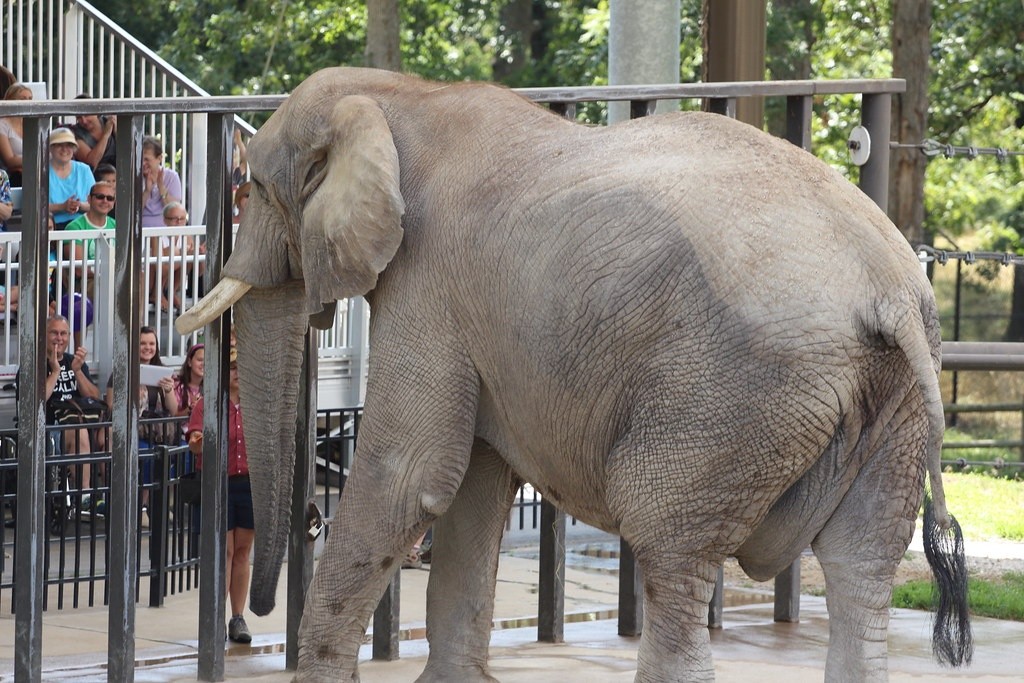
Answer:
[50, 393, 110, 433]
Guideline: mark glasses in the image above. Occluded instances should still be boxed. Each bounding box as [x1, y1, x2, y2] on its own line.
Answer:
[165, 216, 186, 224]
[230, 362, 237, 370]
[91, 193, 115, 202]
[243, 192, 250, 198]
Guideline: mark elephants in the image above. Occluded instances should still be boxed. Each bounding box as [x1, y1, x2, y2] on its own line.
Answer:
[175, 66, 973, 683]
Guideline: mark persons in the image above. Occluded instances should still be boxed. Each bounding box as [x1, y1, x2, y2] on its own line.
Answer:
[17, 215, 58, 316]
[185, 345, 254, 643]
[15, 316, 205, 527]
[64, 182, 116, 297]
[0, 66, 254, 229]
[149, 203, 206, 313]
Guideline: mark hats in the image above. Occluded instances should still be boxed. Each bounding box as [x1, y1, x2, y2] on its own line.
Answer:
[49, 127, 78, 148]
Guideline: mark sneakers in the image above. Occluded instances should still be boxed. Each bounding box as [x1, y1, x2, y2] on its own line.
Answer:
[229, 618, 251, 642]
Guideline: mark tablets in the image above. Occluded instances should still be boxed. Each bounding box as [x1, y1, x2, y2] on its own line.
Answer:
[140, 365, 173, 386]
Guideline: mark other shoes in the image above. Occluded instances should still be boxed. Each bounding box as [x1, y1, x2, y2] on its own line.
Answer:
[142, 511, 149, 527]
[80, 496, 106, 521]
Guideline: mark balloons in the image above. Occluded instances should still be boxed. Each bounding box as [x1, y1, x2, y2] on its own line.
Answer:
[57, 292, 92, 330]
